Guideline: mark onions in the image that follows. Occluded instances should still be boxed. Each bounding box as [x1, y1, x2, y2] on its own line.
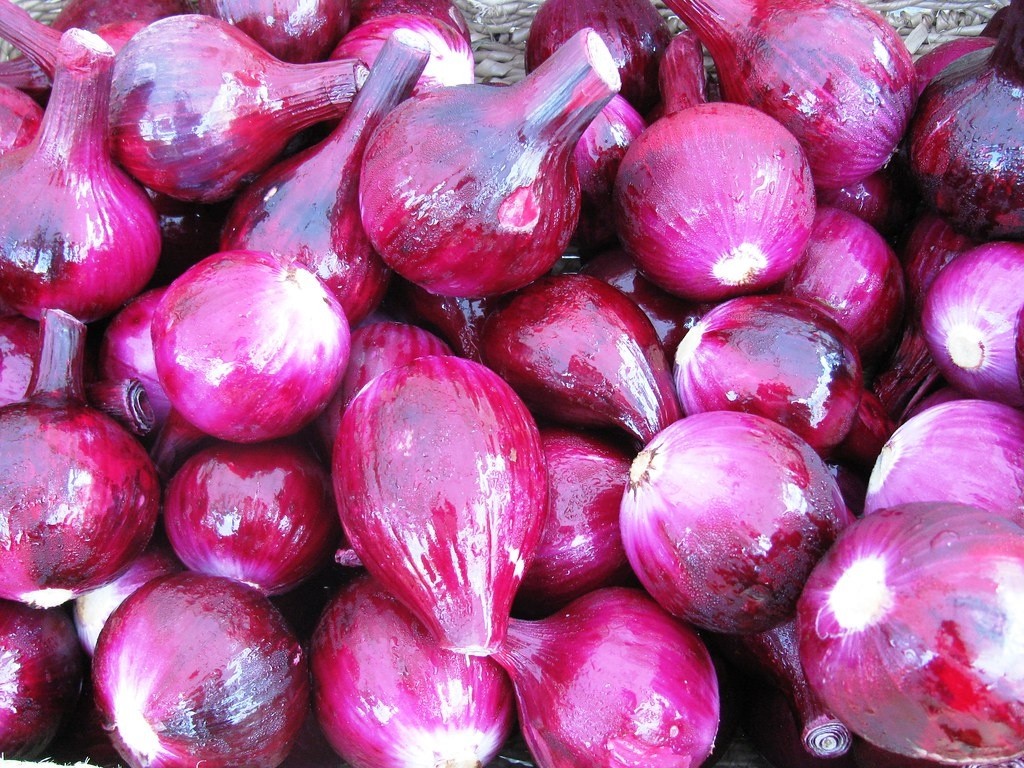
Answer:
[0, 0, 1024, 768]
